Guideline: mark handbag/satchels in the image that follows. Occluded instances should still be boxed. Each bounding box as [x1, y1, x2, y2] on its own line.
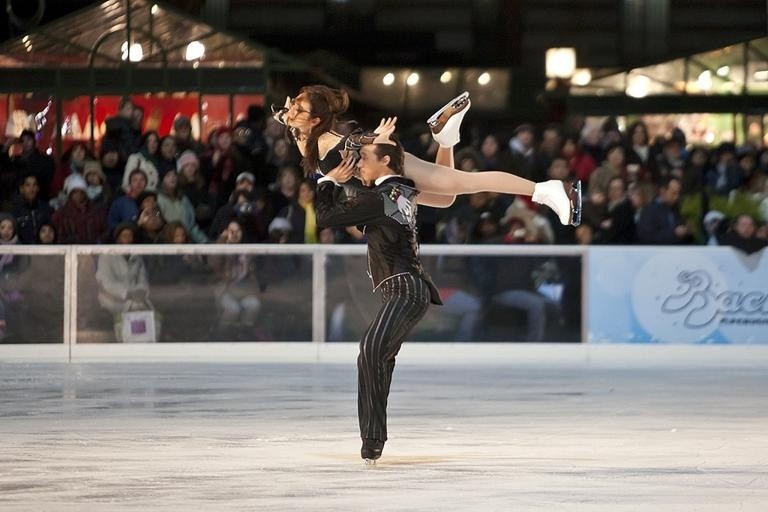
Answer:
[112, 308, 162, 342]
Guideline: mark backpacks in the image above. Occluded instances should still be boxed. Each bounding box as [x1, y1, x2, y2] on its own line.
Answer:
[537, 284, 564, 307]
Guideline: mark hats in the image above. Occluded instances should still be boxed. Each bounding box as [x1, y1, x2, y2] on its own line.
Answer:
[0, 115, 255, 239]
[267, 216, 293, 232]
[702, 210, 726, 226]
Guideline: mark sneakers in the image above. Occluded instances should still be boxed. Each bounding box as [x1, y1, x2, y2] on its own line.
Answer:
[358, 437, 385, 460]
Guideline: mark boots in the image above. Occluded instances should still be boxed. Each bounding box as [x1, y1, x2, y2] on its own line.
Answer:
[532, 178, 575, 226]
[430, 99, 471, 149]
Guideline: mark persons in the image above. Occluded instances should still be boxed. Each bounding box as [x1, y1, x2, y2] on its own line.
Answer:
[271, 84, 578, 227]
[313, 133, 445, 461]
[1, 95, 767, 343]
